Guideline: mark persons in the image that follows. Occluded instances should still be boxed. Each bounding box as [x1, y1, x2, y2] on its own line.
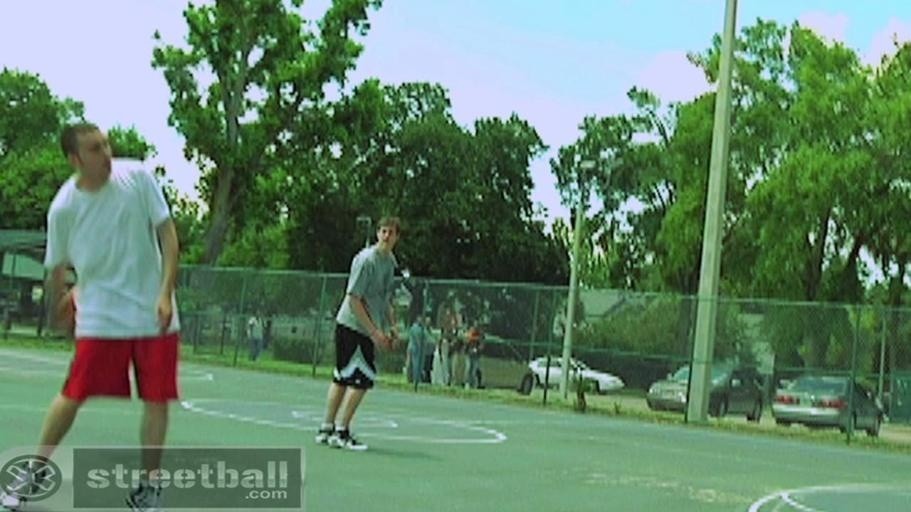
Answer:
[4, 123, 180, 510]
[246, 309, 263, 360]
[407, 316, 485, 388]
[314, 217, 402, 451]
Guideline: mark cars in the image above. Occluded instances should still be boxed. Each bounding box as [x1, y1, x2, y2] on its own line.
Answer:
[407, 326, 910, 438]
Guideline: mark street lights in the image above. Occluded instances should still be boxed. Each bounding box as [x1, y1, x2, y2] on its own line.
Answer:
[553, 154, 603, 401]
[355, 214, 372, 250]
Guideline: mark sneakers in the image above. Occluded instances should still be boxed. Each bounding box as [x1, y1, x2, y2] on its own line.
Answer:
[1, 458, 45, 511]
[314, 422, 335, 444]
[327, 426, 367, 451]
[124, 479, 161, 510]
